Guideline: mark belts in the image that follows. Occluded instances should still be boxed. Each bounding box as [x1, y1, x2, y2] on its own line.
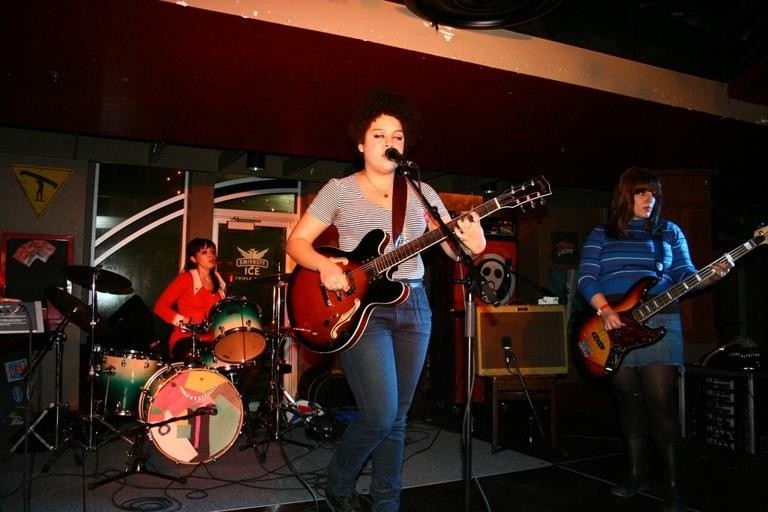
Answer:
[405, 282, 423, 288]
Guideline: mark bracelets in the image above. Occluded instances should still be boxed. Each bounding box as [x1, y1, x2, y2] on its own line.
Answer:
[708, 277, 719, 283]
[594, 300, 610, 316]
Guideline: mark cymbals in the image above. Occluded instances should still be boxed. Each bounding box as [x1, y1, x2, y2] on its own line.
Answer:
[48, 293, 97, 333]
[61, 263, 134, 296]
[250, 271, 293, 285]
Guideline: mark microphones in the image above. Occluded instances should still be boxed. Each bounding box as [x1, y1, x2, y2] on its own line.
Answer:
[503, 332, 513, 368]
[206, 403, 217, 416]
[383, 147, 414, 172]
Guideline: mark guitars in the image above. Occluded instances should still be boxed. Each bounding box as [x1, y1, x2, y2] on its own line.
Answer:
[286, 174, 551, 354]
[571, 225, 768, 379]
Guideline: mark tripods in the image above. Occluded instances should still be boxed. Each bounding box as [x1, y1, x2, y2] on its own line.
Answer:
[239, 256, 315, 452]
[42, 273, 135, 476]
[87, 436, 187, 491]
[2, 339, 90, 461]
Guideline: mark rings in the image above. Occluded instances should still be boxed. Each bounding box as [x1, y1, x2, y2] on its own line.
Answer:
[474, 217, 480, 223]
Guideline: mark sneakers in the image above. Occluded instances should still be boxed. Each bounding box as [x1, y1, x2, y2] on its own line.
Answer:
[325, 495, 354, 512]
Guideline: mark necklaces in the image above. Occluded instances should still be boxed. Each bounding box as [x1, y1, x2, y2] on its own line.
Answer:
[361, 169, 394, 198]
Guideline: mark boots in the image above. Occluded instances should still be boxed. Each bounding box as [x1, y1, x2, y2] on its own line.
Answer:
[661, 442, 680, 508]
[611, 434, 648, 497]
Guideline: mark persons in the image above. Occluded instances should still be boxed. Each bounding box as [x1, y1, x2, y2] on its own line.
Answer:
[276, 93, 489, 511]
[150, 237, 230, 362]
[573, 166, 734, 497]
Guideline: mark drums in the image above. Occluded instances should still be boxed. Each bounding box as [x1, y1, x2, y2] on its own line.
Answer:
[100, 349, 165, 412]
[208, 295, 268, 362]
[137, 361, 245, 464]
[205, 346, 253, 418]
